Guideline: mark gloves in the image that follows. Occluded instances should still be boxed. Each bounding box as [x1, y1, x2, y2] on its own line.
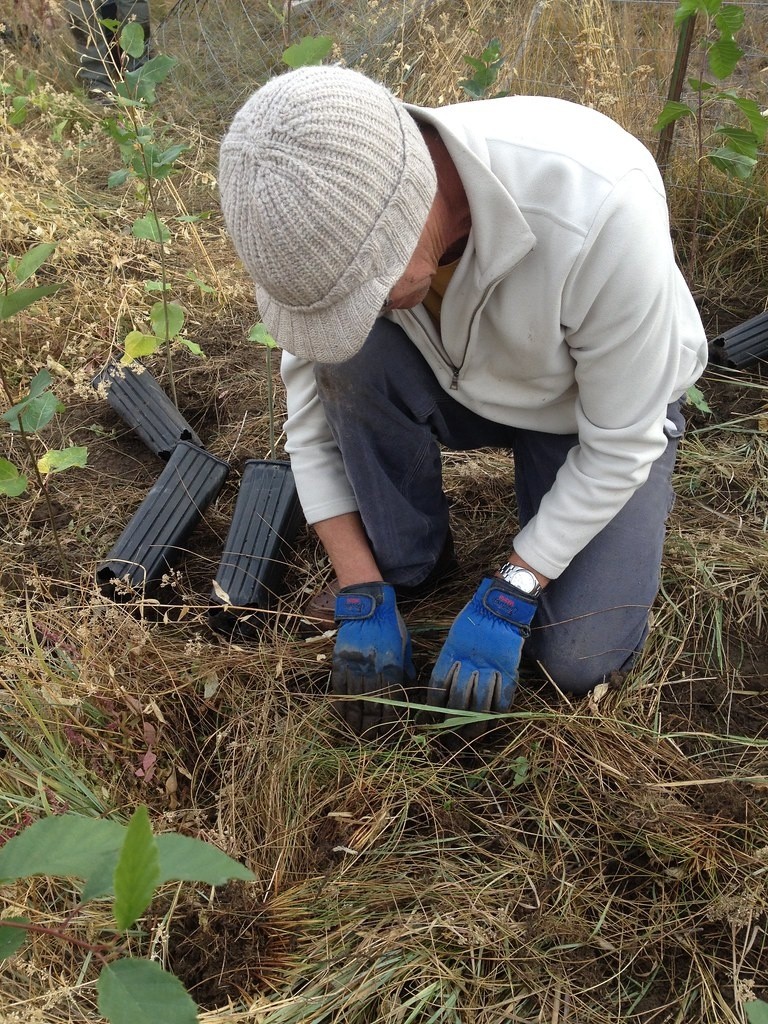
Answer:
[328, 582, 416, 740]
[426, 574, 540, 748]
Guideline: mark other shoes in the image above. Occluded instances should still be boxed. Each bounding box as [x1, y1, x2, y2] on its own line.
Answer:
[302, 532, 460, 641]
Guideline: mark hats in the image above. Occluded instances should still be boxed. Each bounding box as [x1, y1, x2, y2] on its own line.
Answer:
[218, 65, 438, 364]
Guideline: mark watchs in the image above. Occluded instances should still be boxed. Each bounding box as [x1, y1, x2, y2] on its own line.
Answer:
[498, 561, 543, 598]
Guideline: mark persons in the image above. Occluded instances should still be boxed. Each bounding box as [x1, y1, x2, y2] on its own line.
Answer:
[63, 0, 152, 92]
[220, 64, 708, 757]
